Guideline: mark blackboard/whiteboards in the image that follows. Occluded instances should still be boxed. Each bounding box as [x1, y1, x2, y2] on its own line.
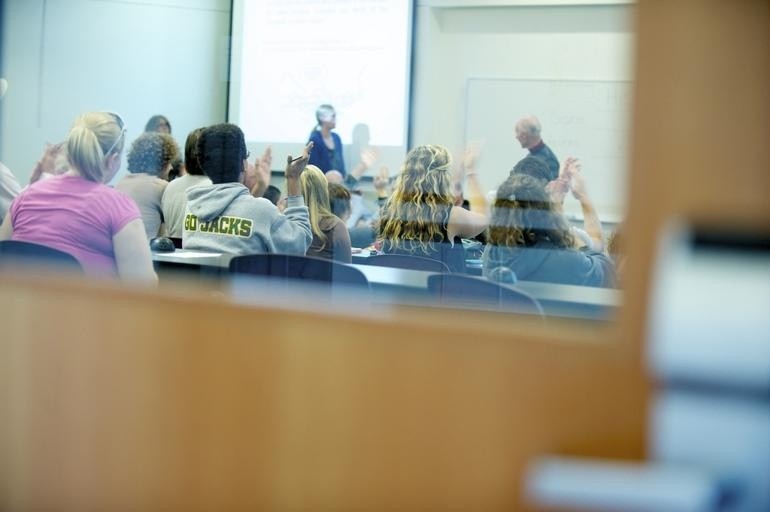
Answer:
[461, 77, 635, 224]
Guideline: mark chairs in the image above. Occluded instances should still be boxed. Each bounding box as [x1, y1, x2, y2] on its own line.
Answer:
[428, 272, 544, 316]
[367, 253, 453, 276]
[229, 254, 372, 298]
[3, 239, 88, 287]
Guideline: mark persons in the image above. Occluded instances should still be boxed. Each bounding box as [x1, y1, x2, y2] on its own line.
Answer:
[375, 145, 486, 270]
[1, 104, 390, 288]
[482, 114, 608, 289]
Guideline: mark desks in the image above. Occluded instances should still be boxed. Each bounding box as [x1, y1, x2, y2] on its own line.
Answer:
[149, 245, 620, 317]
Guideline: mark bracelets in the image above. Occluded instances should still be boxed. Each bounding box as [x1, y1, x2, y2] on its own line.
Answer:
[468, 171, 477, 176]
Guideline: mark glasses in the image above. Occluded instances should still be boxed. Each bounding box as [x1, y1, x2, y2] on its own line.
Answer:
[244, 149, 250, 158]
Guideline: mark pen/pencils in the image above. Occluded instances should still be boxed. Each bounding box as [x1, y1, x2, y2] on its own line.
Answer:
[291, 151, 311, 162]
